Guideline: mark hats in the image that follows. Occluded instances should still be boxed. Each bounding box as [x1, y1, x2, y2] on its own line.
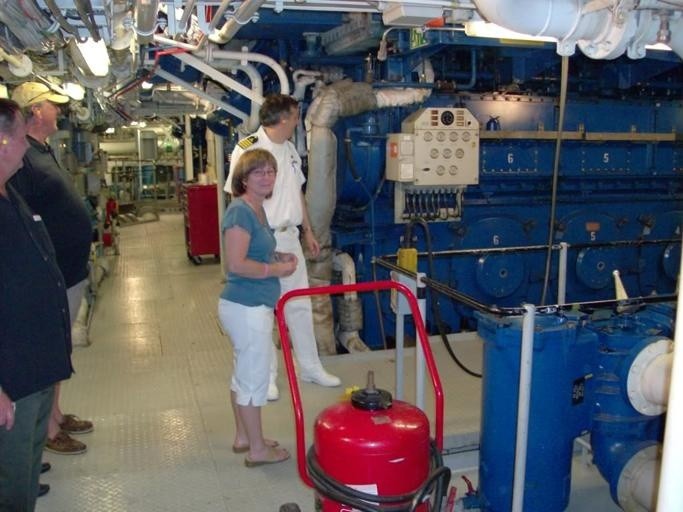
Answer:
[11, 81, 70, 109]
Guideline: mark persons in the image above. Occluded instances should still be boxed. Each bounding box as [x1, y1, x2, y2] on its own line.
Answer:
[215, 147, 292, 468]
[8, 79, 93, 453]
[222, 92, 342, 402]
[1, 95, 76, 510]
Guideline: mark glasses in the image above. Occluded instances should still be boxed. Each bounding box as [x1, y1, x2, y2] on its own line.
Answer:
[247, 168, 276, 178]
[28, 87, 56, 102]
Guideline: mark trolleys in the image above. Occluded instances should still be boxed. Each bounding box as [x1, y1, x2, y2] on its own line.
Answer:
[273, 277, 459, 510]
[181, 181, 219, 267]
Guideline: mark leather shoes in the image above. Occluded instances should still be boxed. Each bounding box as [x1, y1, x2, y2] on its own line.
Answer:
[60, 414, 92, 434]
[40, 463, 50, 473]
[45, 431, 87, 455]
[38, 484, 49, 496]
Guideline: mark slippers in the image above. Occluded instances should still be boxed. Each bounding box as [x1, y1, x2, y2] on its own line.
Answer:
[233, 439, 289, 468]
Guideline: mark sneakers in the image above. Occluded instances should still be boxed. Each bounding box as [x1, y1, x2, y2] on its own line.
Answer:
[300, 372, 341, 387]
[266, 382, 280, 401]
